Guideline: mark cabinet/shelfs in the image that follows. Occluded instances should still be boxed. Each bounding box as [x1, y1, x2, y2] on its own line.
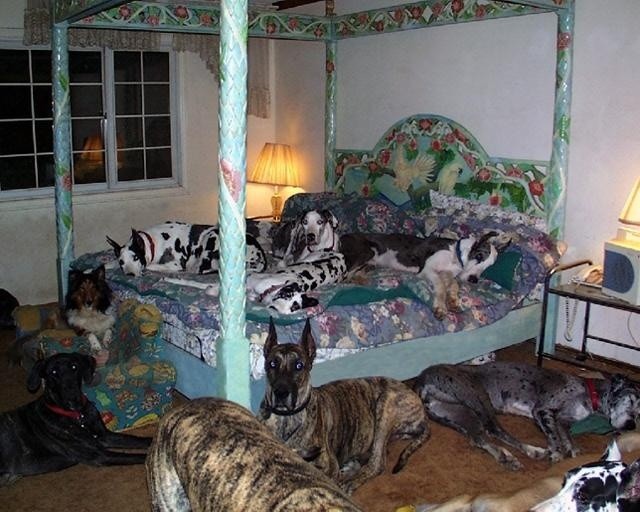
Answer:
[536, 258, 640, 383]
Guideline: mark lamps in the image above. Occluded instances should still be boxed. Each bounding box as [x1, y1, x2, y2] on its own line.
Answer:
[76, 130, 127, 183]
[246, 141, 302, 223]
[617, 177, 640, 248]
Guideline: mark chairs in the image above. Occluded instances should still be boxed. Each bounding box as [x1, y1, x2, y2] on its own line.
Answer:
[12, 299, 179, 436]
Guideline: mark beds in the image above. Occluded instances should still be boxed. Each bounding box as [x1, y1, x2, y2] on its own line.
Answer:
[22, 0, 578, 418]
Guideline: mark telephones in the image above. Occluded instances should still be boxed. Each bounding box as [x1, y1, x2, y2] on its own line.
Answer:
[571, 265, 604, 289]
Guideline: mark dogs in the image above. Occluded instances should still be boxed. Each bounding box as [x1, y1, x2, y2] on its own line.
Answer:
[156, 252, 347, 320]
[336, 230, 514, 323]
[82, 219, 267, 277]
[461, 352, 497, 365]
[143, 395, 367, 512]
[413, 368, 640, 471]
[245, 206, 341, 268]
[527, 432, 640, 512]
[0, 351, 155, 486]
[63, 261, 118, 352]
[256, 313, 432, 496]
